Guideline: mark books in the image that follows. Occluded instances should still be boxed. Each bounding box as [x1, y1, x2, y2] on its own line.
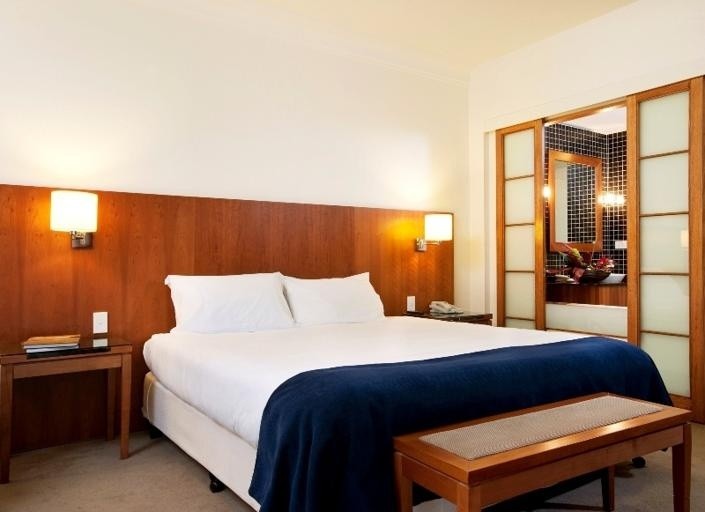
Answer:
[22, 333, 81, 349]
[25, 343, 80, 352]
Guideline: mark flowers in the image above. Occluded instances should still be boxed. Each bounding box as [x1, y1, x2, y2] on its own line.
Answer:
[547, 239, 618, 285]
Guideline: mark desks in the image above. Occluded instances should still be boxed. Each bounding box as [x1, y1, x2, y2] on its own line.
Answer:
[0, 343, 133, 485]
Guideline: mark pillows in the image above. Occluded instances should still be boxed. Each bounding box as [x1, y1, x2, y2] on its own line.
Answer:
[280, 270, 390, 319]
[162, 270, 298, 336]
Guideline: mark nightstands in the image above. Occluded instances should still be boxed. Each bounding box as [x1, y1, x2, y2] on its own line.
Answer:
[402, 310, 494, 326]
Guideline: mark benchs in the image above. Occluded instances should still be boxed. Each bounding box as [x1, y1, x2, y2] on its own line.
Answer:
[390, 389, 695, 511]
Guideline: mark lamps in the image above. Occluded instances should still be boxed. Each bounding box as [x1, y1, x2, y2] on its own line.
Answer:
[49, 187, 100, 250]
[413, 210, 454, 254]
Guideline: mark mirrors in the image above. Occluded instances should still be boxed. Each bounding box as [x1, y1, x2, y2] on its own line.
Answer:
[542, 101, 630, 347]
[547, 148, 606, 255]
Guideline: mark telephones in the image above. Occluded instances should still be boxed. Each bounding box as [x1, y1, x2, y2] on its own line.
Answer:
[430, 301, 464, 314]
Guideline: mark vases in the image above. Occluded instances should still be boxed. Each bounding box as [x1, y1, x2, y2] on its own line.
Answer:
[581, 270, 613, 284]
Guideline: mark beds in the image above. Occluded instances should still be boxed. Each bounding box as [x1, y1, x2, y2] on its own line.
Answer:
[140, 315, 673, 510]
